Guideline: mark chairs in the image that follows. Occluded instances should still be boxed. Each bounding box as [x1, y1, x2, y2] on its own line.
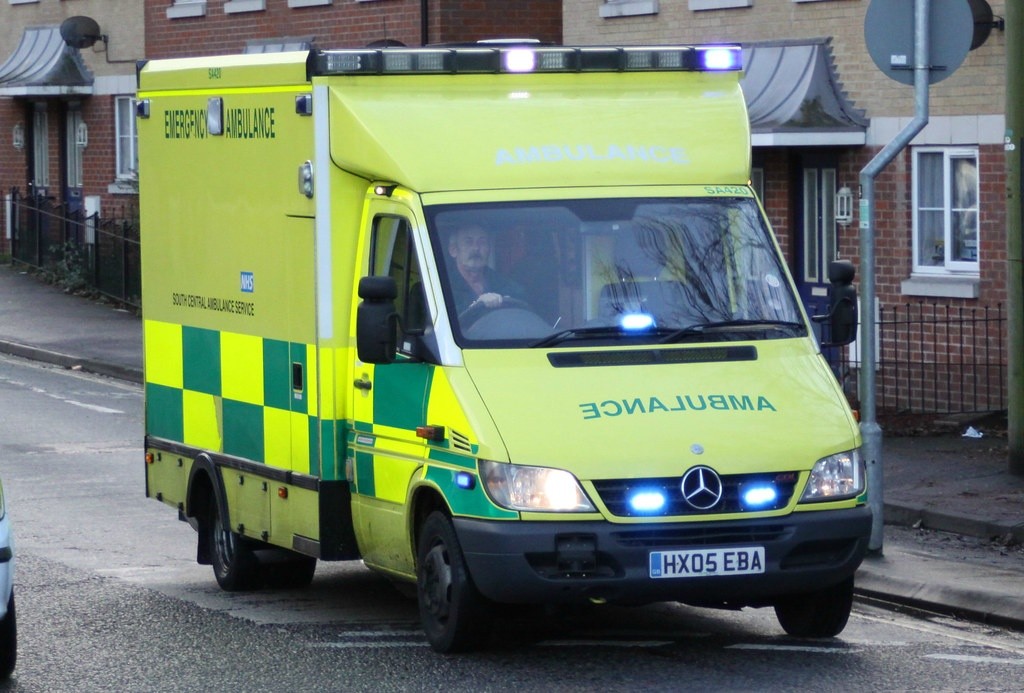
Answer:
[595, 226, 701, 328]
[403, 226, 461, 362]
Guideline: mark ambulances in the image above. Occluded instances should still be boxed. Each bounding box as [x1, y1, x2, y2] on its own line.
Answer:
[134, 38, 869, 656]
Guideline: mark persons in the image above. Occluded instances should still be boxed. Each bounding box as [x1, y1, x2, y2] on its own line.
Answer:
[424, 219, 525, 336]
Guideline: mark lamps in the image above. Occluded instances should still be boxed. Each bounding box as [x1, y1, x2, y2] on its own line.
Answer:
[835, 181, 854, 227]
[75, 120, 88, 149]
[12, 120, 25, 150]
